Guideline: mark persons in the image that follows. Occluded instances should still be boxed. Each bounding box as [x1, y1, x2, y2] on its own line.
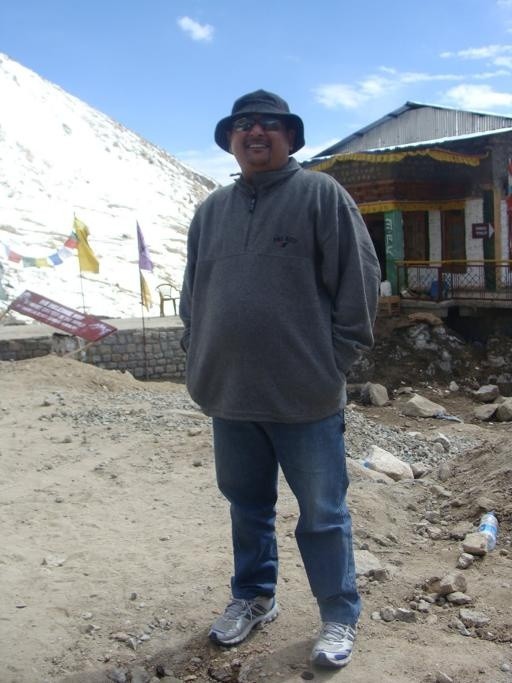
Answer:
[174, 86, 386, 668]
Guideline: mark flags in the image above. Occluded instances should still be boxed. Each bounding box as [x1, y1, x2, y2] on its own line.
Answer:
[58, 246, 73, 257]
[72, 216, 100, 275]
[22, 256, 38, 268]
[48, 252, 64, 266]
[6, 250, 23, 264]
[36, 257, 48, 268]
[64, 235, 81, 248]
[137, 270, 155, 311]
[136, 223, 154, 272]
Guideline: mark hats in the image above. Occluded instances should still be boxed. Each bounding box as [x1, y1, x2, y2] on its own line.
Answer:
[215, 90, 306, 156]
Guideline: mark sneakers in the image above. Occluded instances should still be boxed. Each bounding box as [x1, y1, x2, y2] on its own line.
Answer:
[309, 618, 358, 669]
[207, 590, 279, 645]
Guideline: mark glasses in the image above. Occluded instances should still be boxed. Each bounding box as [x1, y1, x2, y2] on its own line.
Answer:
[229, 115, 280, 132]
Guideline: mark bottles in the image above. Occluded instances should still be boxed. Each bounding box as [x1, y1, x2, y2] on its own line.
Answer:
[476, 511, 499, 552]
